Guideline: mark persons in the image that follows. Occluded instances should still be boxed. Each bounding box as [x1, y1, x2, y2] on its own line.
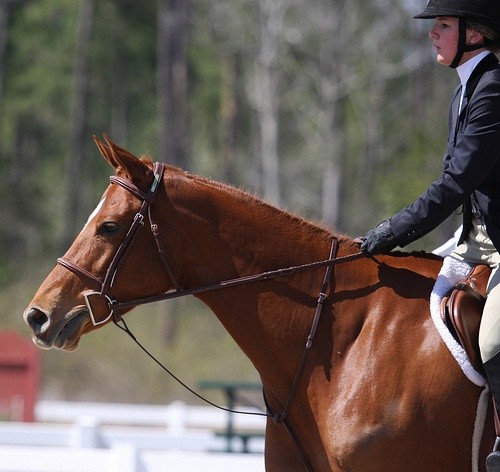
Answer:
[353, 0, 500, 472]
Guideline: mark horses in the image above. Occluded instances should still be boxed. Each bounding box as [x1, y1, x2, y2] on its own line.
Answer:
[22, 131, 500, 472]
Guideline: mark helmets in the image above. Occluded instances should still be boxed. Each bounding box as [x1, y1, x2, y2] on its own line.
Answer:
[413, 0, 500, 33]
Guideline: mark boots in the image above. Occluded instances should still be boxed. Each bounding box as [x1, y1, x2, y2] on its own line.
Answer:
[483, 351, 500, 472]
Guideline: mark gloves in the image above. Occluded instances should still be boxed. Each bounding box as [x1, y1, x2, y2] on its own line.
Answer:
[361, 217, 397, 256]
